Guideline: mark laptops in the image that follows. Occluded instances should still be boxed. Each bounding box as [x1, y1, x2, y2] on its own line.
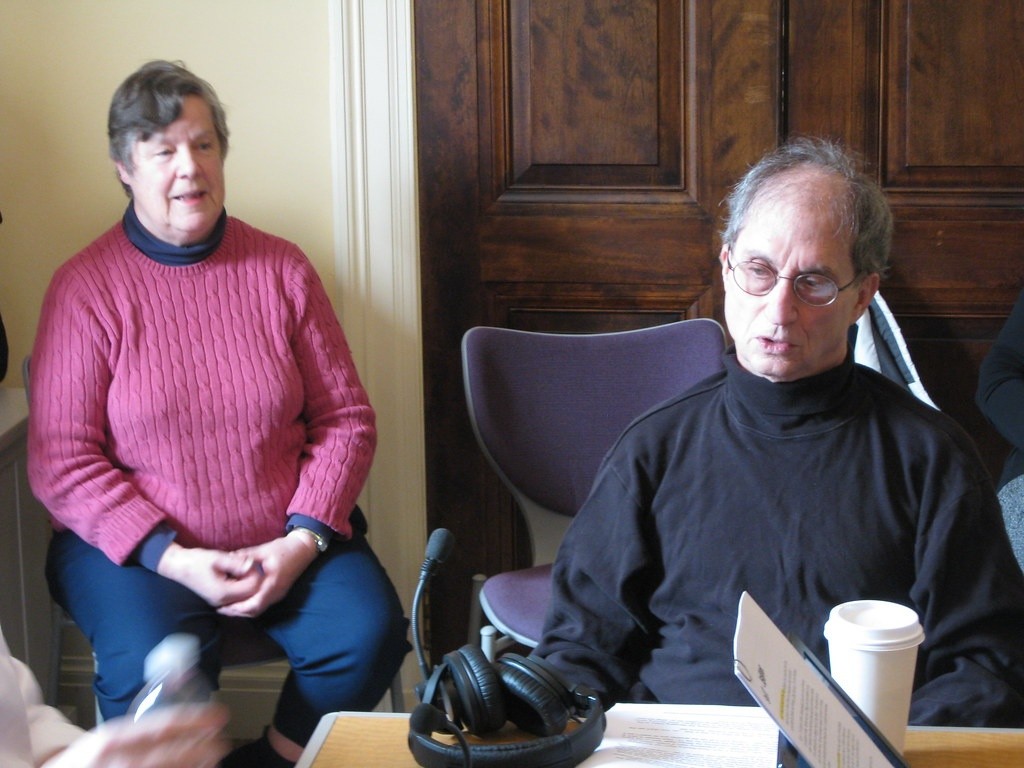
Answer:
[776, 631, 911, 768]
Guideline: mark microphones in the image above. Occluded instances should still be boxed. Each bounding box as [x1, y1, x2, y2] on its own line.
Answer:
[411, 528, 456, 701]
[410, 703, 472, 768]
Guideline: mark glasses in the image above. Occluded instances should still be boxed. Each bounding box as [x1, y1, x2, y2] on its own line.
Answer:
[727, 245, 866, 307]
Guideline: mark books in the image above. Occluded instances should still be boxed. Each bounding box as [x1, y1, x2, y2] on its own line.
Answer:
[572, 701, 780, 767]
[733, 590, 894, 768]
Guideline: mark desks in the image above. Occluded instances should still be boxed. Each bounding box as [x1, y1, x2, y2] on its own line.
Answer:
[294, 703, 1024, 768]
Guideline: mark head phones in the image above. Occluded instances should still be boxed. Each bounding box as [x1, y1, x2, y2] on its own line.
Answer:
[409, 643, 606, 768]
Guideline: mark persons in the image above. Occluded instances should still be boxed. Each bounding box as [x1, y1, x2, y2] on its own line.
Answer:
[971, 280, 1024, 576]
[1, 624, 233, 768]
[528, 147, 1024, 728]
[27, 63, 410, 768]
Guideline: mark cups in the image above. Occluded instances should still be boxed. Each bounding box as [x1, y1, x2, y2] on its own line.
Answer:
[823, 600, 926, 758]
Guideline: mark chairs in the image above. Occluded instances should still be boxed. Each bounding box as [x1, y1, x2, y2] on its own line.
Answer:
[461, 317, 728, 664]
[220, 620, 412, 715]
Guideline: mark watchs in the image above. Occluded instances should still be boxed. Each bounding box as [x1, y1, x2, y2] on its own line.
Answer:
[292, 527, 328, 554]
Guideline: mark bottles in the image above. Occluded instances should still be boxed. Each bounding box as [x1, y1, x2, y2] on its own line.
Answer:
[127, 632, 210, 720]
